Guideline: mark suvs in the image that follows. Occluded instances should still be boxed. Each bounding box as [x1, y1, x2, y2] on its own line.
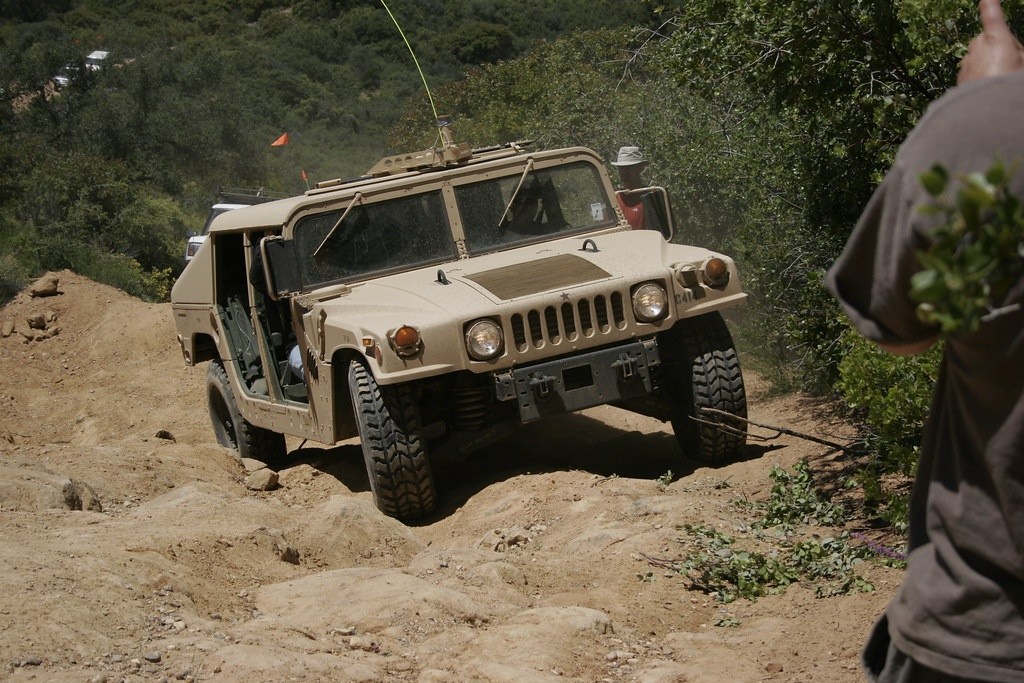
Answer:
[170, 139, 751, 523]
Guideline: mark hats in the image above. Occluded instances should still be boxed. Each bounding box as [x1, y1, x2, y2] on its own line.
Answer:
[610, 145, 649, 166]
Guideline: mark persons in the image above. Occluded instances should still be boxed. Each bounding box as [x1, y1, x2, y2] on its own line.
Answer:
[824, 0, 1024, 683]
[598, 147, 651, 230]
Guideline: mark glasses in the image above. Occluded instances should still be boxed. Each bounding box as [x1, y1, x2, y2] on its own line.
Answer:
[512, 197, 539, 206]
